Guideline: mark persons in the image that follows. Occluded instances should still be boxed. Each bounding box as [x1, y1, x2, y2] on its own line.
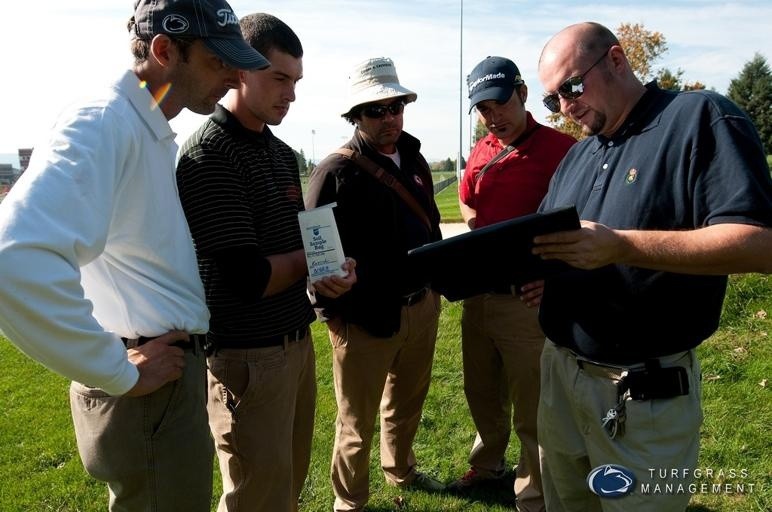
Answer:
[300, 55, 446, 511]
[170, 12, 360, 511]
[529, 19, 772, 511]
[2, 0, 273, 511]
[446, 55, 580, 510]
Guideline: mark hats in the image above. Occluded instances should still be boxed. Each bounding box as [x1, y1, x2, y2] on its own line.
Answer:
[467, 56, 524, 114]
[127, 0, 271, 73]
[340, 56, 417, 124]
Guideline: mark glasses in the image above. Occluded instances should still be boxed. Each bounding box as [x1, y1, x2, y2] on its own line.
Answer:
[361, 102, 404, 119]
[542, 50, 607, 113]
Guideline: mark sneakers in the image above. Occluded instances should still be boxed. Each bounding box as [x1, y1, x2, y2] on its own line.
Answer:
[403, 471, 446, 494]
[446, 464, 506, 489]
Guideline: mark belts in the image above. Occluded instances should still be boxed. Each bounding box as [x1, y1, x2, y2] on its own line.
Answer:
[488, 284, 524, 296]
[538, 340, 693, 382]
[402, 288, 428, 308]
[119, 336, 206, 359]
[212, 326, 311, 349]
